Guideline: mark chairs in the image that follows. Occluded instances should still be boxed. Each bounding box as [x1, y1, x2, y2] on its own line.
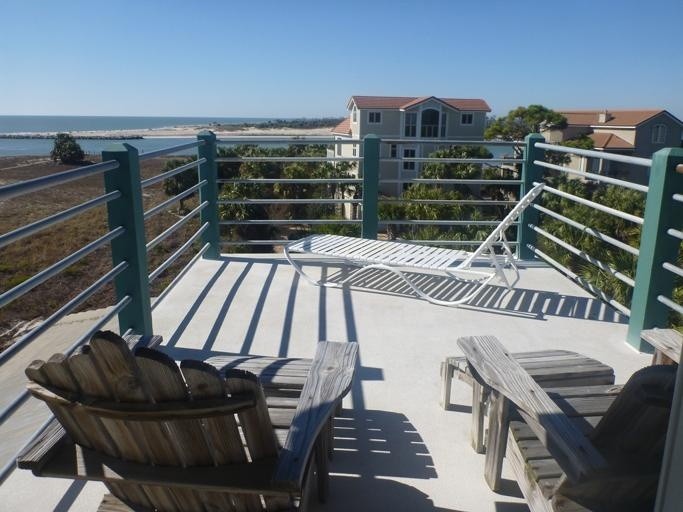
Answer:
[14, 328, 360, 511]
[439, 328, 683, 511]
[283, 182, 546, 307]
